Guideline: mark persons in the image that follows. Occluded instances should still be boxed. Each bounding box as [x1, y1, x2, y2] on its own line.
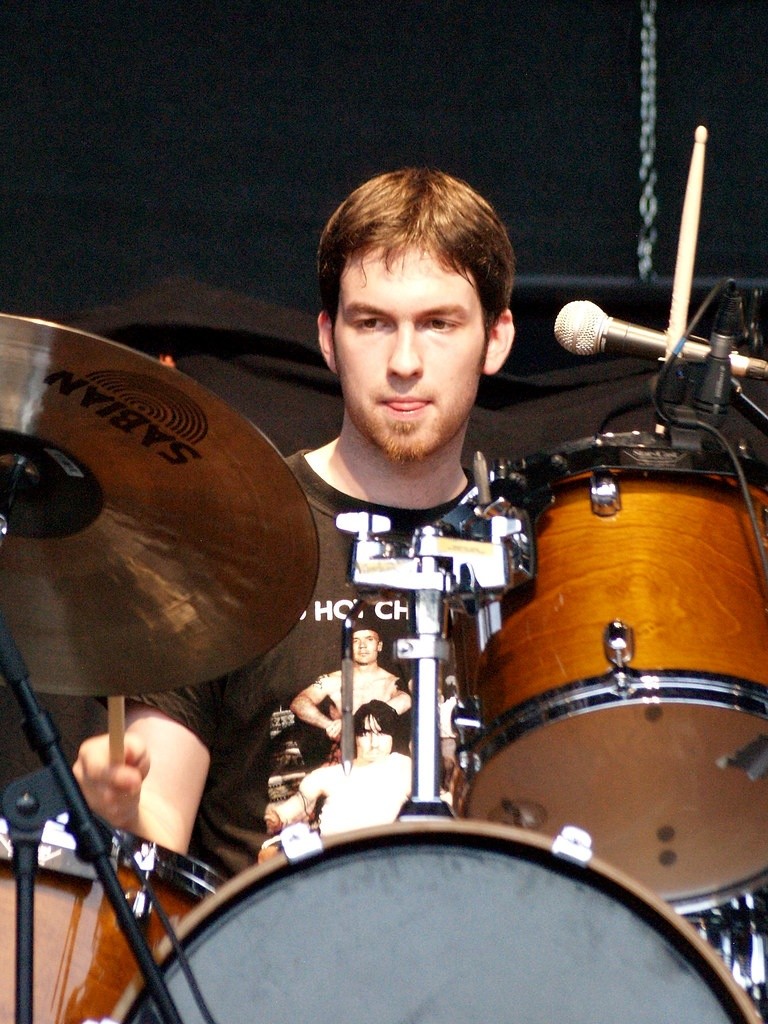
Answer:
[69, 168, 539, 881]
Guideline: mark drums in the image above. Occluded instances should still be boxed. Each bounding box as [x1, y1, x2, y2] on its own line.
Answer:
[0, 807, 231, 1024]
[99, 816, 763, 1024]
[451, 425, 768, 918]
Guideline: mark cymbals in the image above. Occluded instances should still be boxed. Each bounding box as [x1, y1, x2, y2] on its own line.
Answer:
[1, 309, 320, 698]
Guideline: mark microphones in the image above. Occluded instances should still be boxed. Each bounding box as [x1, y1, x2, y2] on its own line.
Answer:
[554, 300, 768, 380]
[692, 280, 743, 429]
